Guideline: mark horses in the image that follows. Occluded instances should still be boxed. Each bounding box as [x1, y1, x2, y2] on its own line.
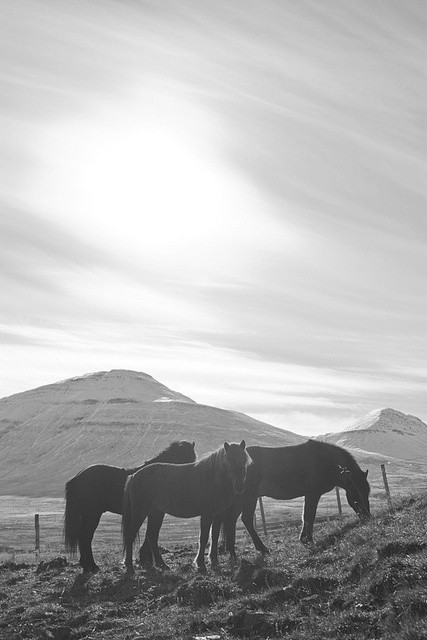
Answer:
[217, 439, 371, 556]
[63, 438, 199, 574]
[120, 440, 254, 575]
